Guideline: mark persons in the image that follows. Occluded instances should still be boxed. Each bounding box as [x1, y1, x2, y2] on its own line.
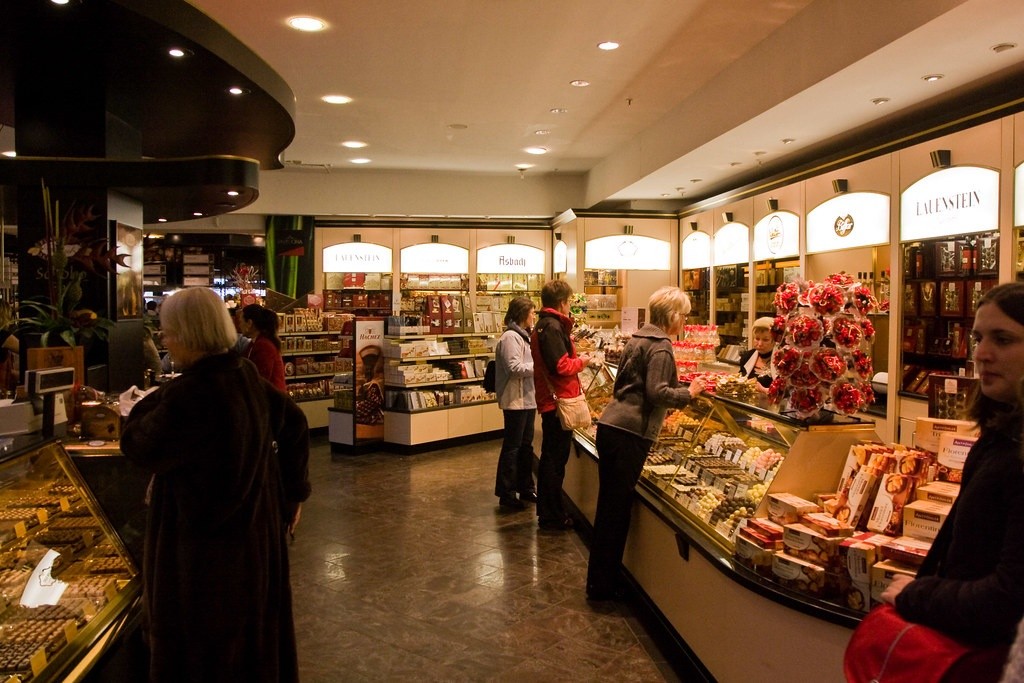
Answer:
[240, 304, 287, 392]
[0, 299, 19, 398]
[224, 292, 251, 354]
[143, 333, 179, 374]
[495, 297, 538, 509]
[146, 301, 159, 315]
[122, 286, 311, 683]
[738, 316, 776, 392]
[882, 282, 1024, 683]
[584, 285, 708, 600]
[531, 280, 594, 531]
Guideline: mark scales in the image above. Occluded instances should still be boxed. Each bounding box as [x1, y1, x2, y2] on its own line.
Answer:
[24, 363, 128, 477]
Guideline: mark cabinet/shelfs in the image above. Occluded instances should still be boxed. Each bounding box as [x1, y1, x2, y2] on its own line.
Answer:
[278, 316, 383, 446]
[580, 227, 1002, 548]
[381, 334, 504, 448]
[322, 274, 549, 334]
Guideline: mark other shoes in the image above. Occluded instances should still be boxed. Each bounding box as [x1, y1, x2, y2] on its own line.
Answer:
[538, 516, 574, 529]
[519, 491, 538, 503]
[589, 591, 625, 601]
[499, 491, 524, 509]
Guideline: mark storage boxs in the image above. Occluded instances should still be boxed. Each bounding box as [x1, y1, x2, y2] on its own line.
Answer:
[735, 418, 980, 614]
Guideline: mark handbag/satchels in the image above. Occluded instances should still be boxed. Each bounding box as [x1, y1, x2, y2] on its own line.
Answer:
[843, 596, 1010, 683]
[482, 361, 496, 393]
[557, 393, 592, 431]
